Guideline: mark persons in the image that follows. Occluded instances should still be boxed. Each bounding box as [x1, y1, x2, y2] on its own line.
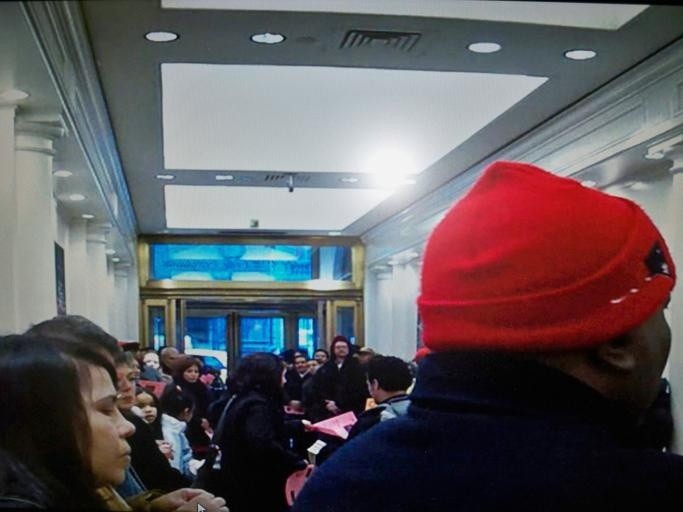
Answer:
[277, 335, 413, 470]
[1, 333, 231, 511]
[287, 162, 683, 511]
[215, 353, 312, 511]
[111, 342, 226, 499]
[21, 315, 149, 502]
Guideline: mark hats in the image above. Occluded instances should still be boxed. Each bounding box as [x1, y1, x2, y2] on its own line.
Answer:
[417, 161, 677, 352]
[357, 347, 375, 356]
